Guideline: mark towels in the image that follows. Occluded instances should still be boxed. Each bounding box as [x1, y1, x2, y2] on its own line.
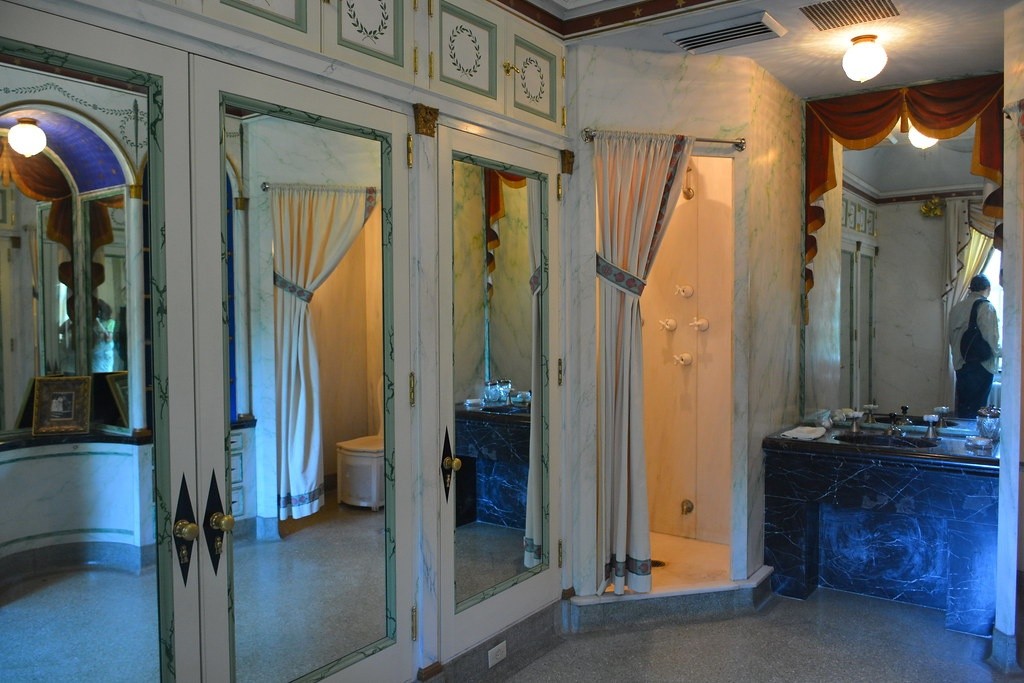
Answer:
[780, 426, 827, 441]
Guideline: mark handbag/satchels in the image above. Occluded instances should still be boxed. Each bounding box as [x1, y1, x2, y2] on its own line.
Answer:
[960, 299, 994, 363]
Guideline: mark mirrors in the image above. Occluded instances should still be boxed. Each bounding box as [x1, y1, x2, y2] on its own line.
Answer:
[219, 86, 402, 683]
[78, 186, 131, 439]
[0, 33, 176, 683]
[840, 248, 874, 412]
[0, 127, 79, 440]
[34, 204, 78, 378]
[800, 73, 1007, 437]
[452, 144, 549, 613]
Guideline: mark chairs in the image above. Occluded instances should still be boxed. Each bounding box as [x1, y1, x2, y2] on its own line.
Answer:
[335, 376, 385, 511]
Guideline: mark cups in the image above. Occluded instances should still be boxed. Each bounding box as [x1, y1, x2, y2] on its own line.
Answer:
[977, 407, 1001, 442]
[499, 380, 512, 401]
[484, 379, 499, 401]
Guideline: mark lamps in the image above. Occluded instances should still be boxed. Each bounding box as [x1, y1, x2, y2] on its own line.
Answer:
[7, 116, 52, 158]
[908, 125, 940, 150]
[838, 30, 888, 82]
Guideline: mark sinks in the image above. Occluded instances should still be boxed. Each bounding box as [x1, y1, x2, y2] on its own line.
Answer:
[833, 430, 944, 449]
[874, 416, 958, 428]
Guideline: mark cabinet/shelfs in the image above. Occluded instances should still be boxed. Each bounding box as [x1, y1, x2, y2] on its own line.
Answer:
[140, 0, 567, 137]
[841, 189, 878, 238]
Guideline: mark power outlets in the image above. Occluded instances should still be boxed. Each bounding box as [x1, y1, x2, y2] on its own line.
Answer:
[487, 640, 507, 669]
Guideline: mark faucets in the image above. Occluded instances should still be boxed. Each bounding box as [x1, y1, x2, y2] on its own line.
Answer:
[885, 413, 903, 436]
[896, 406, 912, 425]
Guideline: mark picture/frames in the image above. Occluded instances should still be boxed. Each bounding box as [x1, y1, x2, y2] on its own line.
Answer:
[106, 372, 130, 429]
[31, 373, 92, 438]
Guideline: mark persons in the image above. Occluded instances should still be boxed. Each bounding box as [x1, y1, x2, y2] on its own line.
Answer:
[949, 275, 999, 418]
[91, 300, 115, 372]
